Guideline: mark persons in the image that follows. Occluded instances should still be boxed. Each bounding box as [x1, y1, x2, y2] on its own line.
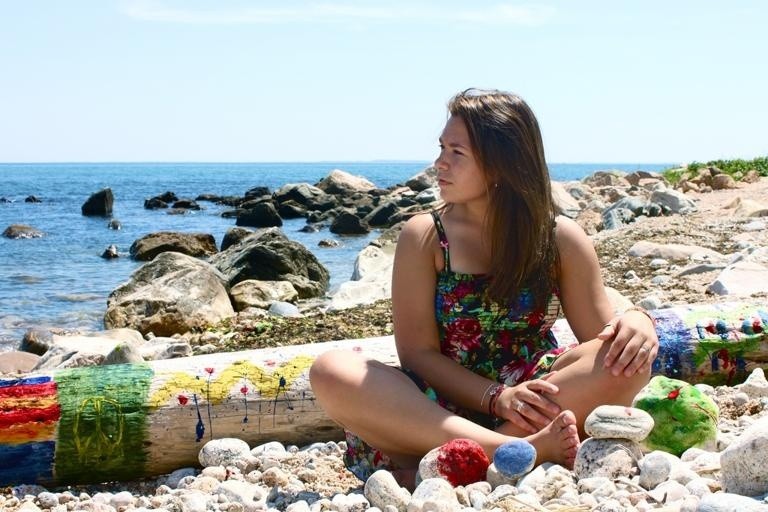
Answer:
[308, 88, 659, 473]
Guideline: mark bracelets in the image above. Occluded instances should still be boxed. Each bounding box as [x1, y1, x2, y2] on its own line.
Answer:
[480, 384, 506, 420]
[625, 305, 658, 327]
